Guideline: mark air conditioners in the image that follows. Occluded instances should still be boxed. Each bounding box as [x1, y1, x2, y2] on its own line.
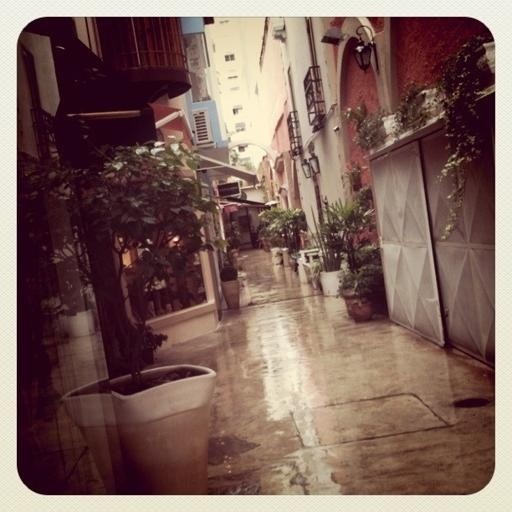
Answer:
[193, 109, 216, 150]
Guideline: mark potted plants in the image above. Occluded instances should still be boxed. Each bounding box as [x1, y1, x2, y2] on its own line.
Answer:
[17, 142, 218, 492]
[47, 253, 96, 338]
[381, 112, 404, 140]
[258, 198, 383, 321]
[396, 85, 443, 130]
[442, 36, 496, 241]
[220, 267, 239, 310]
[346, 107, 382, 147]
[225, 242, 242, 270]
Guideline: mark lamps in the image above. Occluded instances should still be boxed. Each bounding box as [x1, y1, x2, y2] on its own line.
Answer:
[308, 150, 321, 176]
[300, 158, 312, 180]
[352, 25, 379, 74]
